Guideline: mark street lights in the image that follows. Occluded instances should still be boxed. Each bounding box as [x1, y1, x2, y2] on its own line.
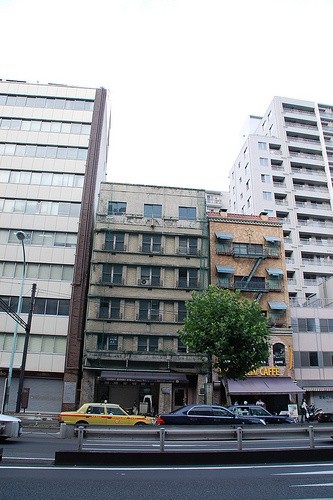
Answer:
[1, 231, 25, 415]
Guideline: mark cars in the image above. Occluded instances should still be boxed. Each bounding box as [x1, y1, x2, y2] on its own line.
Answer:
[227, 404, 297, 424]
[156, 404, 265, 426]
[58, 403, 161, 438]
[318, 412, 333, 423]
[0, 415, 23, 442]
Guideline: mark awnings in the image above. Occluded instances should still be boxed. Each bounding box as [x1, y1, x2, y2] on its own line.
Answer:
[227, 377, 305, 395]
[101, 371, 187, 383]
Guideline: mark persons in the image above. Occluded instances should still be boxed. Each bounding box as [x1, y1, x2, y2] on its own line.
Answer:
[87, 407, 113, 415]
[302, 398, 315, 422]
[132, 400, 156, 418]
[234, 399, 265, 415]
